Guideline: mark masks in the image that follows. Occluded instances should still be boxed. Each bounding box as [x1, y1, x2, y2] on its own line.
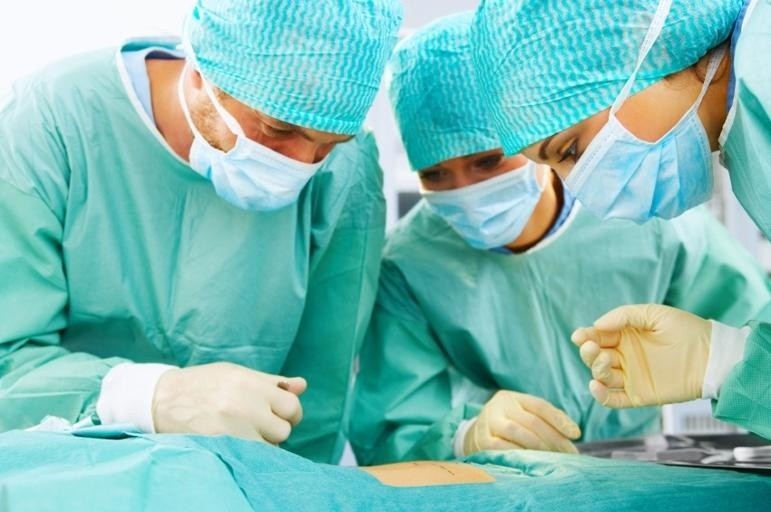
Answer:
[562, 1, 733, 225]
[179, 59, 332, 212]
[419, 161, 542, 251]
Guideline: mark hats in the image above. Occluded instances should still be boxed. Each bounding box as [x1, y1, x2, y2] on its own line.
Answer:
[182, 0, 404, 136]
[387, 8, 502, 172]
[470, 0, 745, 158]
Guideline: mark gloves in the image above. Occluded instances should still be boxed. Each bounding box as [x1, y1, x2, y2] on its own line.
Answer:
[571, 303, 712, 409]
[463, 389, 582, 455]
[154, 361, 307, 449]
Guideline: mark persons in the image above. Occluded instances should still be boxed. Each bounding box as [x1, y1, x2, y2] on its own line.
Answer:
[340, 12, 771, 461]
[1, 1, 390, 468]
[468, 0, 771, 442]
